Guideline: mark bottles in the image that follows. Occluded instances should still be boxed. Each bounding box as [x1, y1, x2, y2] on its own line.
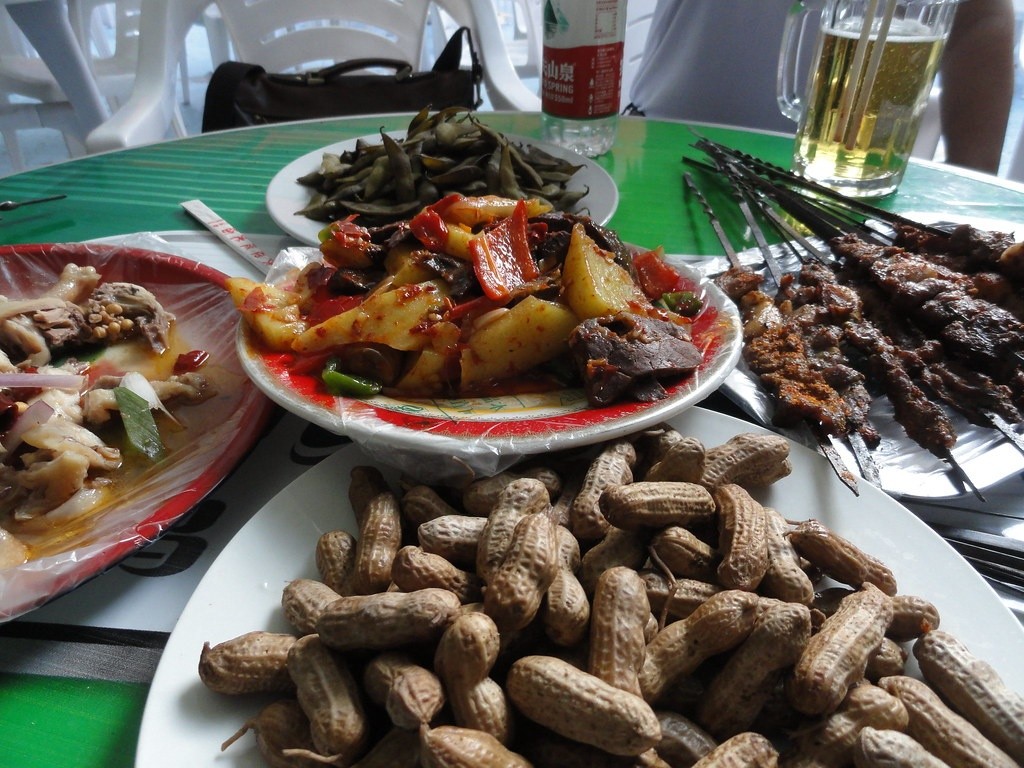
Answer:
[541, 3, 630, 157]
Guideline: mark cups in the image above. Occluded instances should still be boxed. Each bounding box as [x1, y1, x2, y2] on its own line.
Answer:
[779, 0, 964, 200]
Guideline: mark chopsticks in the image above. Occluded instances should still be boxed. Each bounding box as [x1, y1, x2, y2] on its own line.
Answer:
[963, 555, 1024, 587]
[938, 528, 1024, 571]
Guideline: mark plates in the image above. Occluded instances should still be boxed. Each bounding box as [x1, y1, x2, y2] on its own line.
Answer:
[701, 229, 1024, 504]
[0, 239, 268, 636]
[229, 248, 740, 458]
[261, 124, 621, 254]
[135, 399, 1024, 768]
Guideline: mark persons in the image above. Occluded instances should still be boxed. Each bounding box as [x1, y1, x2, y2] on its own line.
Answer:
[626, 0, 1018, 181]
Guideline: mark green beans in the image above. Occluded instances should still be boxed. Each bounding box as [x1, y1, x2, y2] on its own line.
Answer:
[293, 98, 590, 217]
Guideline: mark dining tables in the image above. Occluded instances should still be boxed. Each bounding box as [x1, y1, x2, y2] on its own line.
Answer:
[0, 112, 1024, 768]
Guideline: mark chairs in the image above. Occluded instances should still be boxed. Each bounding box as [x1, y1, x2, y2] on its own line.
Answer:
[1, 0, 542, 179]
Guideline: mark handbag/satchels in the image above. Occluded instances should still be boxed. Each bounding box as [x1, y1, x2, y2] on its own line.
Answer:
[201, 26, 484, 135]
